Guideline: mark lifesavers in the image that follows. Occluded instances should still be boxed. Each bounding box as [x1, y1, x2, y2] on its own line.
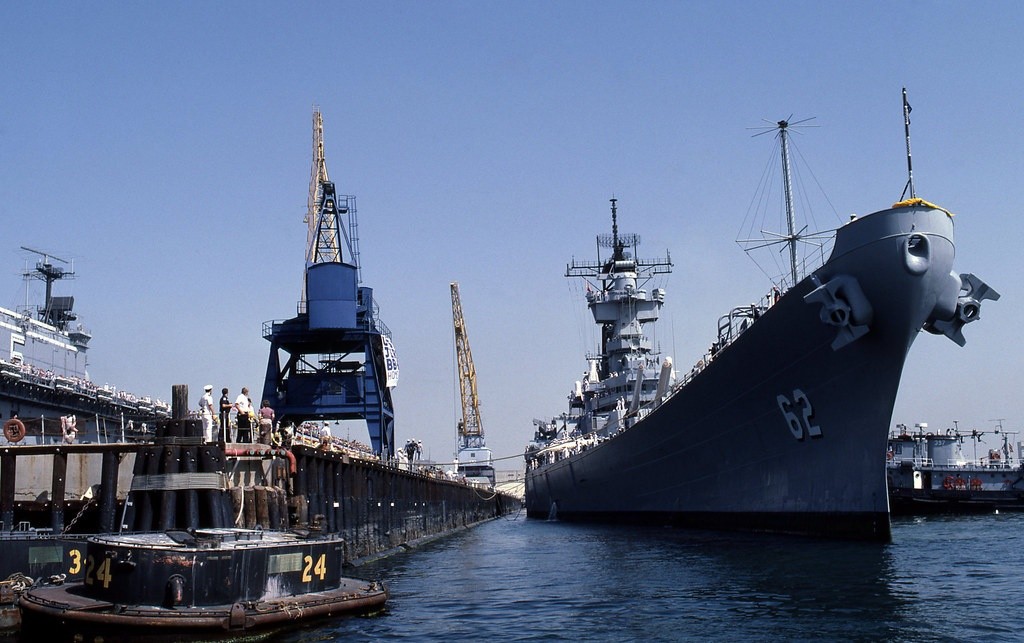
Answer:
[993, 454, 1001, 459]
[943, 476, 954, 488]
[886, 453, 892, 460]
[954, 479, 967, 491]
[970, 479, 983, 491]
[4, 418, 26, 443]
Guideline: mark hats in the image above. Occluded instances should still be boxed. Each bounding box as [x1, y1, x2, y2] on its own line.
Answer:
[418, 440, 422, 443]
[412, 438, 415, 441]
[324, 421, 329, 424]
[203, 384, 213, 390]
[407, 439, 410, 442]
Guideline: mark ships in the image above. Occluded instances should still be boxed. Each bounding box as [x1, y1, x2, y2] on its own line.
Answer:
[524, 113, 1002, 545]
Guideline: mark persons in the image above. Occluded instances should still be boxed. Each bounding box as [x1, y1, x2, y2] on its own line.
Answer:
[766, 293, 773, 308]
[218, 388, 234, 443]
[320, 421, 333, 454]
[773, 285, 781, 304]
[751, 303, 761, 321]
[235, 387, 254, 442]
[198, 385, 217, 441]
[404, 438, 424, 461]
[259, 400, 275, 421]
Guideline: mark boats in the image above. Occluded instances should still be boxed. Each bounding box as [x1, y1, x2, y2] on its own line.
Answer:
[18, 441, 389, 634]
[885, 421, 1024, 501]
